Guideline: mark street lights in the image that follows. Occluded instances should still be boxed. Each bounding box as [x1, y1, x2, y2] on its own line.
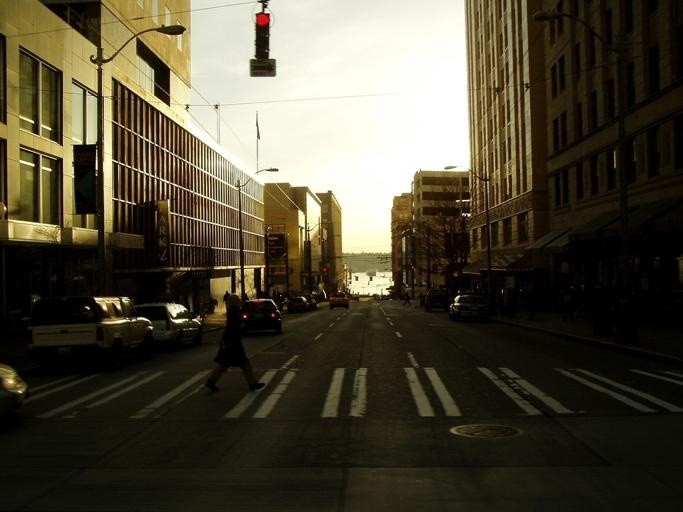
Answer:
[444, 165, 492, 288]
[535, 9, 635, 339]
[305, 219, 334, 290]
[90, 23, 186, 294]
[400, 219, 430, 291]
[236, 167, 278, 297]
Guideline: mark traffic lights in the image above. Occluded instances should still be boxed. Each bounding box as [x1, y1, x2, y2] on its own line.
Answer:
[322, 267, 329, 273]
[255, 13, 270, 58]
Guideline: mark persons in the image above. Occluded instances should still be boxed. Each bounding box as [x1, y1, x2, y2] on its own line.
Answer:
[528, 286, 537, 320]
[561, 287, 575, 323]
[204, 294, 265, 393]
[223, 291, 230, 306]
[402, 292, 411, 306]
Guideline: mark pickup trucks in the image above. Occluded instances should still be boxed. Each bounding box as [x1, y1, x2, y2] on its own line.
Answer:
[28, 296, 154, 365]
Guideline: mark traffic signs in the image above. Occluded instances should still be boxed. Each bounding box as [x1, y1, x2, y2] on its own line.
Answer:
[250, 58, 275, 76]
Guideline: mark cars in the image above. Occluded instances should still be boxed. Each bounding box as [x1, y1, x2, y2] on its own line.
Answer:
[448, 294, 490, 324]
[287, 291, 360, 313]
[133, 301, 202, 356]
[424, 288, 451, 313]
[238, 299, 283, 337]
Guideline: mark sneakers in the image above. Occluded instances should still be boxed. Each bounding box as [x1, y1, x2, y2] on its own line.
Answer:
[205, 382, 221, 392]
[249, 383, 265, 391]
